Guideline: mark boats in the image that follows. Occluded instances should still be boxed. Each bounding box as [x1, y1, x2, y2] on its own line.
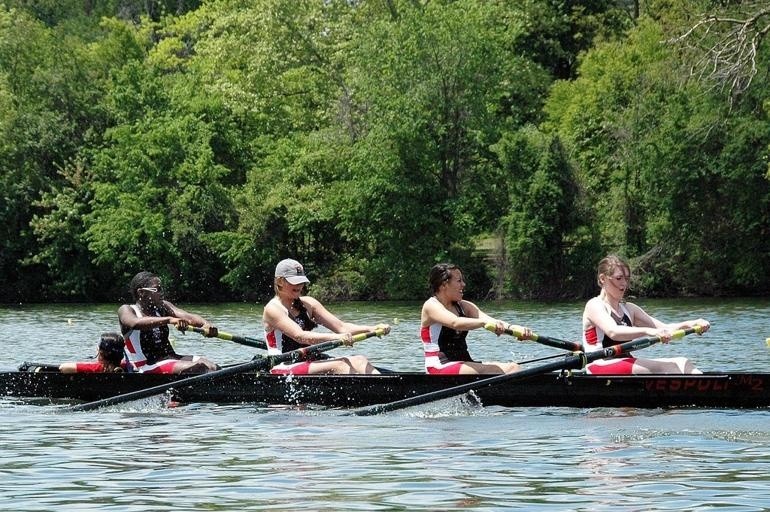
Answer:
[1, 356, 770, 415]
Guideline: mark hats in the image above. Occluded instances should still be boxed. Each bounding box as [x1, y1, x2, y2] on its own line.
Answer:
[276, 259, 312, 285]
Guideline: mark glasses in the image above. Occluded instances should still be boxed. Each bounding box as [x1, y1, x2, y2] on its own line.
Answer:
[141, 286, 162, 294]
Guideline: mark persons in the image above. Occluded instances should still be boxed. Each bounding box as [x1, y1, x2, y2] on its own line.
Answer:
[262, 258, 391, 375]
[87, 332, 127, 374]
[419, 264, 533, 375]
[583, 255, 711, 374]
[117, 271, 221, 375]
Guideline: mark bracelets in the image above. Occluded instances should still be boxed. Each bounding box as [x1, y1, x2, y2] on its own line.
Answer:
[508, 324, 513, 329]
